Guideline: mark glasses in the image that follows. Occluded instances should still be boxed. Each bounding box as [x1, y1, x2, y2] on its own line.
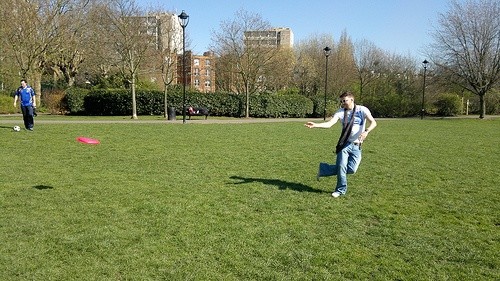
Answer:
[340, 99, 351, 103]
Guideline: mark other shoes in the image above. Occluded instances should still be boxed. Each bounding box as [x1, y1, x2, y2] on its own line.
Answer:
[331, 192, 342, 198]
[317, 163, 323, 181]
[28, 128, 33, 130]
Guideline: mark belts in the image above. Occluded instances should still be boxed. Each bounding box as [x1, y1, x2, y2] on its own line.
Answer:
[349, 142, 358, 145]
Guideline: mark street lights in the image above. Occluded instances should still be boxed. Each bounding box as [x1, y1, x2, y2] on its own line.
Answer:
[421, 59, 429, 120]
[324, 47, 332, 121]
[178, 10, 189, 123]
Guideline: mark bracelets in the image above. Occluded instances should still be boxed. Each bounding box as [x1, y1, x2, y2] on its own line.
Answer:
[365, 130, 367, 132]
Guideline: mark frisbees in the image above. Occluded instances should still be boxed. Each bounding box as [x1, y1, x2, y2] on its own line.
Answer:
[78, 137, 100, 145]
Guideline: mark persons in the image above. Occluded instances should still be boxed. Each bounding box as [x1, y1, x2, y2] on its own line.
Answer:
[14, 80, 37, 131]
[304, 91, 376, 198]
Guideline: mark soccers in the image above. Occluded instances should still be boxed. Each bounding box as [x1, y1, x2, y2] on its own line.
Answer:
[13, 126, 21, 132]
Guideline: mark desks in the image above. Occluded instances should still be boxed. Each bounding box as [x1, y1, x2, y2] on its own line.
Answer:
[184, 106, 209, 120]
